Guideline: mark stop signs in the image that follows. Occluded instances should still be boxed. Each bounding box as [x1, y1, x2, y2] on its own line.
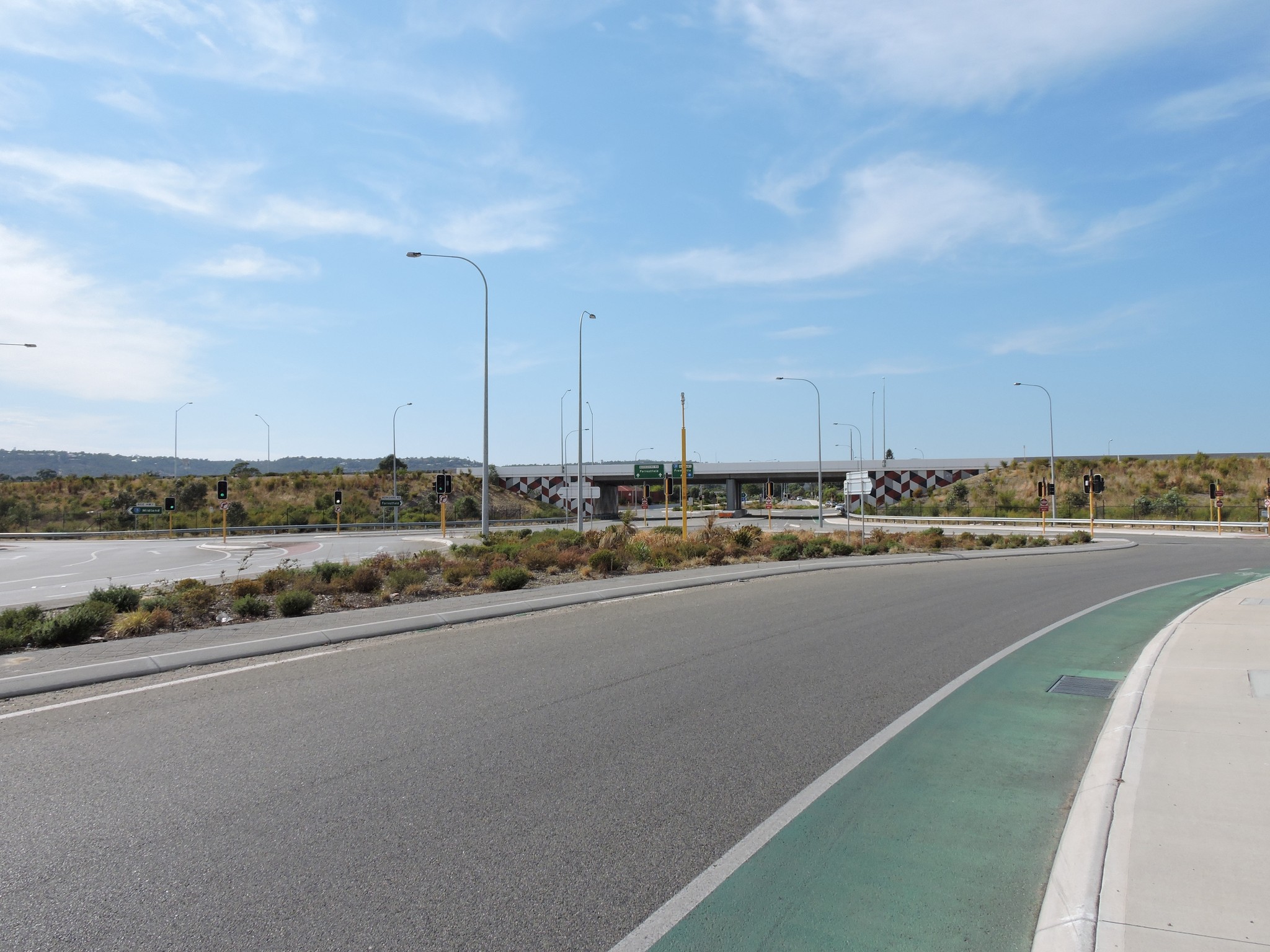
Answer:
[1040, 499, 1048, 505]
[1214, 500, 1223, 508]
[642, 499, 647, 504]
[1264, 500, 1270, 507]
[766, 497, 771, 504]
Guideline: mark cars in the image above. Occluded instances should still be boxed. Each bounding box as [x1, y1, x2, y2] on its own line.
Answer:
[835, 504, 845, 510]
[791, 496, 796, 500]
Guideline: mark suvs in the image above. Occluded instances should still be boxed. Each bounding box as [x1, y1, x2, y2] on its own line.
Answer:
[797, 496, 803, 501]
[825, 501, 838, 508]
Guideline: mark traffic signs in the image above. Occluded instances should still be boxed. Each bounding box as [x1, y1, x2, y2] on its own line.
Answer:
[126, 505, 162, 515]
[382, 496, 401, 500]
[634, 464, 664, 478]
[672, 464, 694, 478]
[380, 500, 403, 506]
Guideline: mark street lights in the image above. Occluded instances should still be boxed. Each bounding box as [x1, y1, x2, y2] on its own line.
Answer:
[1014, 383, 1058, 526]
[635, 447, 654, 516]
[833, 422, 865, 546]
[579, 310, 597, 533]
[586, 401, 594, 465]
[694, 451, 702, 512]
[750, 460, 764, 502]
[1109, 439, 1112, 454]
[776, 377, 823, 528]
[883, 377, 885, 460]
[565, 428, 589, 529]
[406, 251, 490, 539]
[255, 415, 269, 473]
[394, 403, 412, 526]
[872, 391, 875, 459]
[561, 389, 572, 466]
[914, 448, 924, 459]
[175, 402, 193, 478]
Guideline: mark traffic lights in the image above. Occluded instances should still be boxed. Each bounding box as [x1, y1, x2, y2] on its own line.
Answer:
[1209, 483, 1216, 499]
[664, 477, 673, 495]
[165, 497, 175, 510]
[444, 475, 452, 494]
[1047, 483, 1055, 496]
[436, 475, 445, 494]
[1093, 474, 1104, 494]
[766, 482, 774, 496]
[217, 480, 228, 500]
[1267, 487, 1270, 497]
[1038, 482, 1046, 497]
[1083, 475, 1094, 493]
[335, 491, 342, 505]
[643, 485, 650, 498]
[699, 495, 702, 500]
